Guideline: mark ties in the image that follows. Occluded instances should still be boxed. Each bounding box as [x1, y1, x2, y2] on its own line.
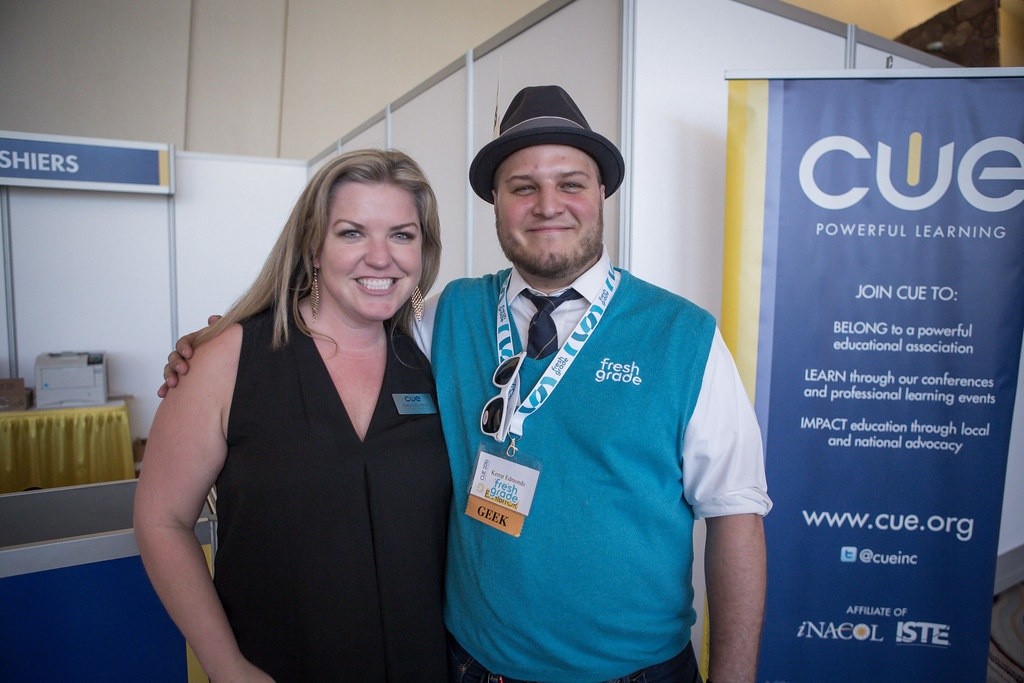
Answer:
[520, 288, 584, 360]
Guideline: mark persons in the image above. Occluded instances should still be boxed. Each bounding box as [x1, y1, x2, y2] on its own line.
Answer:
[130, 147, 454, 681]
[158, 82, 772, 682]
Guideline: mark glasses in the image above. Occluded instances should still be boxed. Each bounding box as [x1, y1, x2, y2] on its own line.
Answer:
[480, 351, 526, 442]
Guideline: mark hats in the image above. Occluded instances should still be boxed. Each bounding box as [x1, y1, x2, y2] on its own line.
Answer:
[469, 85, 625, 205]
